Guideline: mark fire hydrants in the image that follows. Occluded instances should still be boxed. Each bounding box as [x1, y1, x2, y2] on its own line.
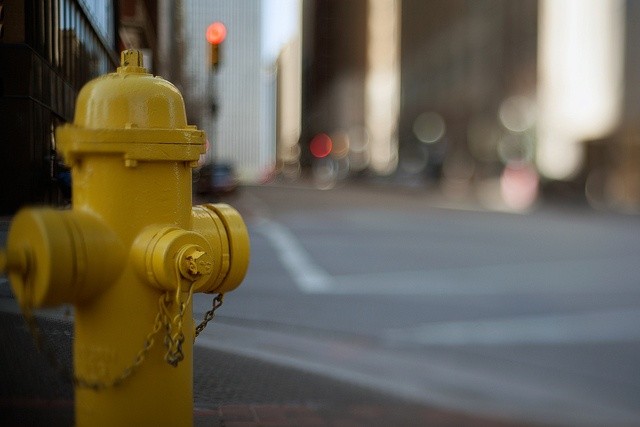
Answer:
[0, 45, 252, 426]
